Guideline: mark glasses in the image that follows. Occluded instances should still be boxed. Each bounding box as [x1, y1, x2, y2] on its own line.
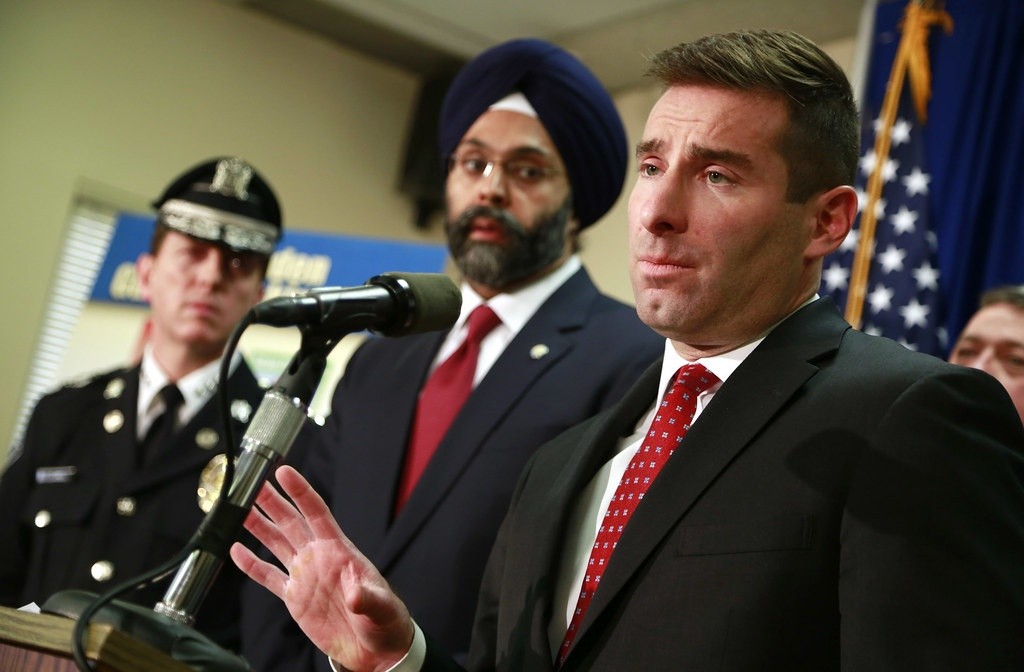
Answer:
[446, 151, 566, 187]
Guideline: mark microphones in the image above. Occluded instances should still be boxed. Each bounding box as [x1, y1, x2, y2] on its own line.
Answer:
[253, 271, 462, 337]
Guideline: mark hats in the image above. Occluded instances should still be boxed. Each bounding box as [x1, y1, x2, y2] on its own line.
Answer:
[439, 39, 630, 236]
[151, 156, 284, 256]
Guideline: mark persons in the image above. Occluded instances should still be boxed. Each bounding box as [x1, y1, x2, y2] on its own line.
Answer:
[240, 36, 667, 672]
[230, 27, 1023, 672]
[945, 284, 1023, 424]
[0, 155, 319, 608]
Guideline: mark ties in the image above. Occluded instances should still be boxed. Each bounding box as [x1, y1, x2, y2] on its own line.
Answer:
[132, 384, 183, 480]
[391, 304, 505, 526]
[556, 366, 718, 664]
